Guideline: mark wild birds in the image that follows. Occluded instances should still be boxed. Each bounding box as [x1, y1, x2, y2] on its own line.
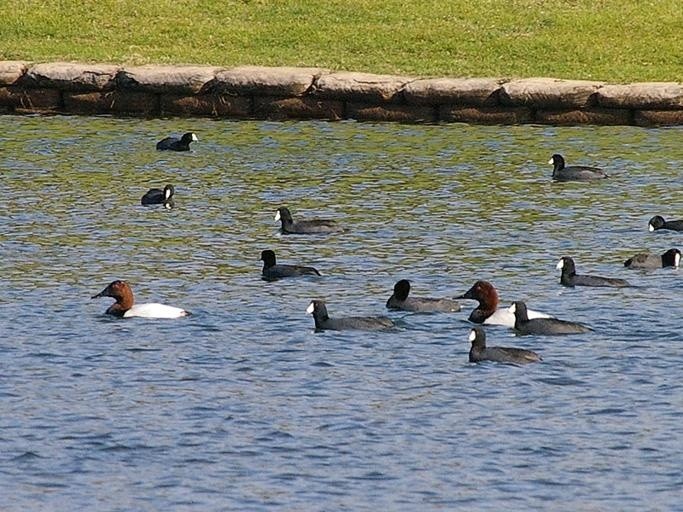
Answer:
[257, 249, 322, 278]
[452, 280, 558, 329]
[555, 256, 631, 287]
[304, 299, 394, 332]
[90, 280, 193, 319]
[648, 215, 683, 232]
[468, 327, 541, 365]
[274, 206, 345, 234]
[624, 248, 681, 269]
[548, 154, 609, 181]
[506, 301, 595, 337]
[385, 279, 461, 313]
[156, 132, 198, 152]
[141, 184, 174, 203]
